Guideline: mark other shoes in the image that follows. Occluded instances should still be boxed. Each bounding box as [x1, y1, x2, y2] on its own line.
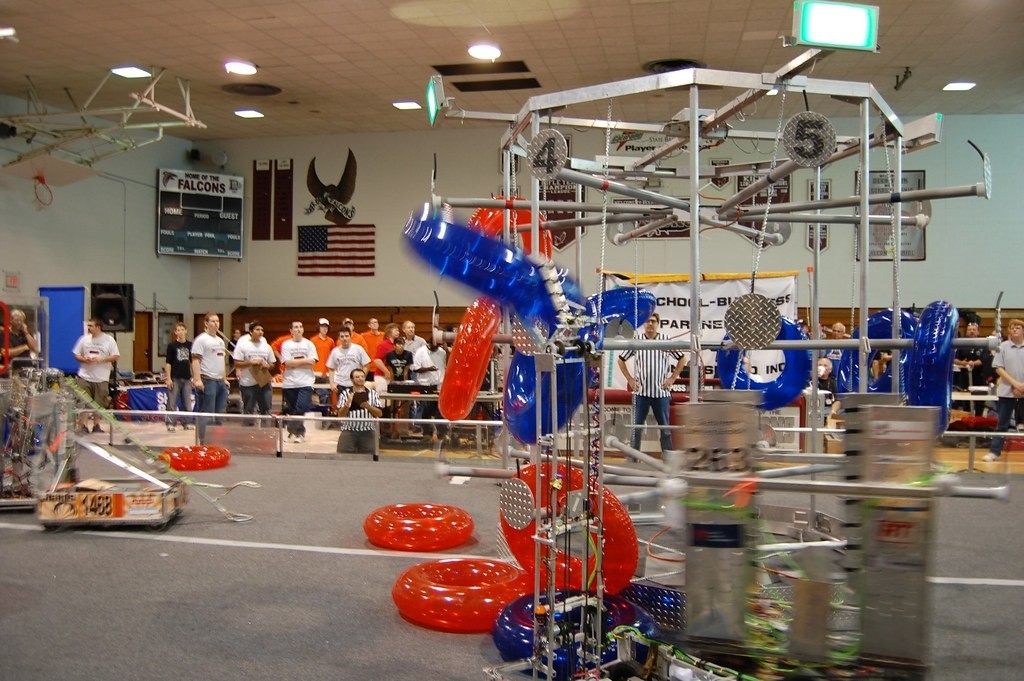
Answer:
[92, 424, 104, 432]
[283, 425, 292, 443]
[294, 434, 306, 442]
[80, 425, 89, 433]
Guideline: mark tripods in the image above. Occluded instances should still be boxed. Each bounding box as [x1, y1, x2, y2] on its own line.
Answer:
[106, 332, 133, 412]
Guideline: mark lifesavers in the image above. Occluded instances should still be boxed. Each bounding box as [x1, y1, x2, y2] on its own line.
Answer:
[717, 316, 812, 413]
[463, 195, 554, 263]
[492, 592, 660, 681]
[402, 212, 585, 325]
[438, 297, 500, 422]
[158, 445, 230, 472]
[390, 556, 535, 631]
[498, 462, 637, 594]
[362, 500, 477, 551]
[909, 299, 960, 438]
[834, 308, 917, 405]
[581, 286, 656, 349]
[503, 297, 605, 444]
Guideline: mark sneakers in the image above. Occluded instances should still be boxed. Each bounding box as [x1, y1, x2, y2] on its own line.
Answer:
[981, 452, 1001, 462]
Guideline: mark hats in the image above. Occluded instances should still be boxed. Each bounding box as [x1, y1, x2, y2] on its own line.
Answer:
[319, 317, 330, 325]
[343, 318, 354, 326]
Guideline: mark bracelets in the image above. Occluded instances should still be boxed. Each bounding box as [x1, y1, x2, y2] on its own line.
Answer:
[24, 331, 29, 335]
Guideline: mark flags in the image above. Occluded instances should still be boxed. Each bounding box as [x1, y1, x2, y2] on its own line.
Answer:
[295, 223, 377, 278]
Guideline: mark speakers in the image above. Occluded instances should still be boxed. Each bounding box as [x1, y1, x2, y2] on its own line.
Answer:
[90, 283, 134, 333]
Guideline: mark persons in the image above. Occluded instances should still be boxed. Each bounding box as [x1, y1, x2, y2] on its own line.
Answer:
[226, 306, 1024, 463]
[189, 312, 230, 446]
[163, 322, 195, 434]
[70, 319, 120, 434]
[0, 309, 38, 378]
